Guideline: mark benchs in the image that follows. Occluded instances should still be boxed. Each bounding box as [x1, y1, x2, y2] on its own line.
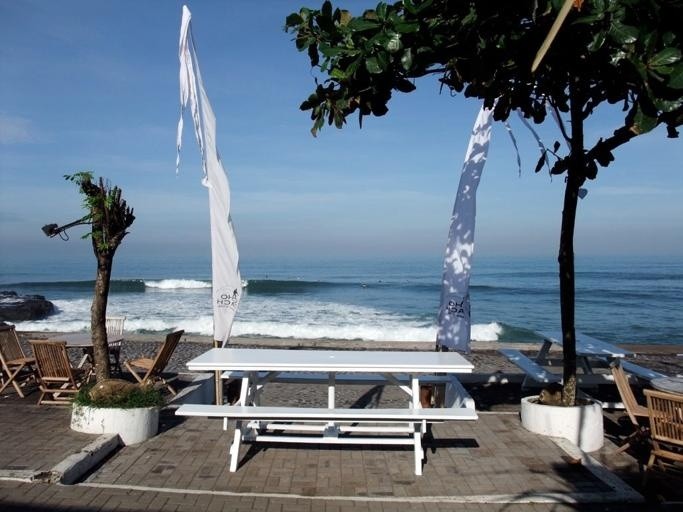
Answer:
[174, 372, 480, 476]
[500, 350, 667, 398]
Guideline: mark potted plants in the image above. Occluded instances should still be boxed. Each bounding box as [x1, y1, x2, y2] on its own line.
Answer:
[284, 1, 683, 453]
[41, 172, 166, 444]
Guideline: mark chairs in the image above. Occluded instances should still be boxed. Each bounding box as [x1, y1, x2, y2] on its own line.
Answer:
[1, 315, 185, 407]
[607, 359, 682, 478]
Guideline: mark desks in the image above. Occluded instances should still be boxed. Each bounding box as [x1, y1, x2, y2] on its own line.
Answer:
[186, 347, 475, 477]
[521, 331, 636, 396]
